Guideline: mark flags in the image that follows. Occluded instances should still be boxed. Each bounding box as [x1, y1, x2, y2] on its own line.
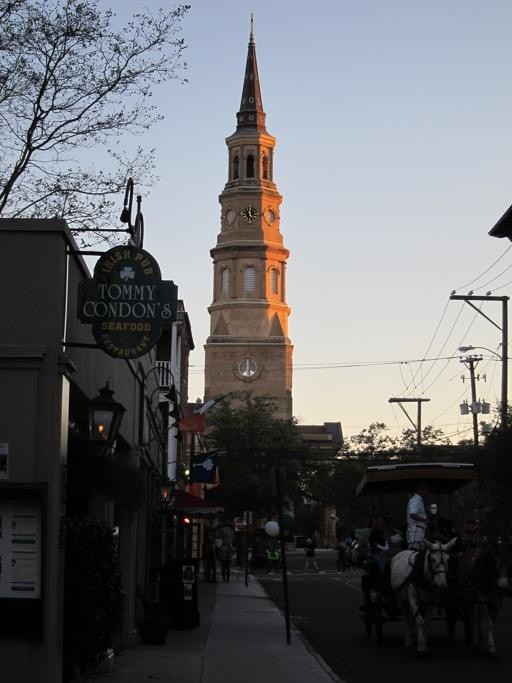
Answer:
[179, 415, 204, 432]
[189, 451, 218, 485]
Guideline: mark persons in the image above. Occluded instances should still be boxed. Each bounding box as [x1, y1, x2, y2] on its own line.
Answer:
[336, 483, 467, 621]
[204, 535, 218, 583]
[304, 537, 320, 572]
[218, 539, 233, 584]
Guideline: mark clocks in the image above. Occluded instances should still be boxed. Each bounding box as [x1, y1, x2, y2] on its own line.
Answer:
[234, 354, 262, 383]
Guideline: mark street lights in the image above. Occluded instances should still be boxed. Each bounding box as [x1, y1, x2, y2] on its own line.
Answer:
[262, 519, 291, 646]
[458, 345, 501, 359]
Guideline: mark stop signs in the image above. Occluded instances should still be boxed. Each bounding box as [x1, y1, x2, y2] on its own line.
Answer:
[462, 518, 481, 536]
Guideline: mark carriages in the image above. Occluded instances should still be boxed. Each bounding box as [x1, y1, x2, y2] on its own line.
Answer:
[357, 462, 509, 661]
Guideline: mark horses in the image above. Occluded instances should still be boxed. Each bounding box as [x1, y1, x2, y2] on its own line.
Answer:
[384, 537, 457, 661]
[335, 542, 352, 572]
[446, 535, 510, 662]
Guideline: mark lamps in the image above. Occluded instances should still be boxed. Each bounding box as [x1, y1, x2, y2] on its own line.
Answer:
[81, 382, 127, 450]
[143, 367, 179, 403]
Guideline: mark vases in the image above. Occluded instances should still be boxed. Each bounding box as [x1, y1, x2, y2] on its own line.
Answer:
[135, 620, 172, 645]
